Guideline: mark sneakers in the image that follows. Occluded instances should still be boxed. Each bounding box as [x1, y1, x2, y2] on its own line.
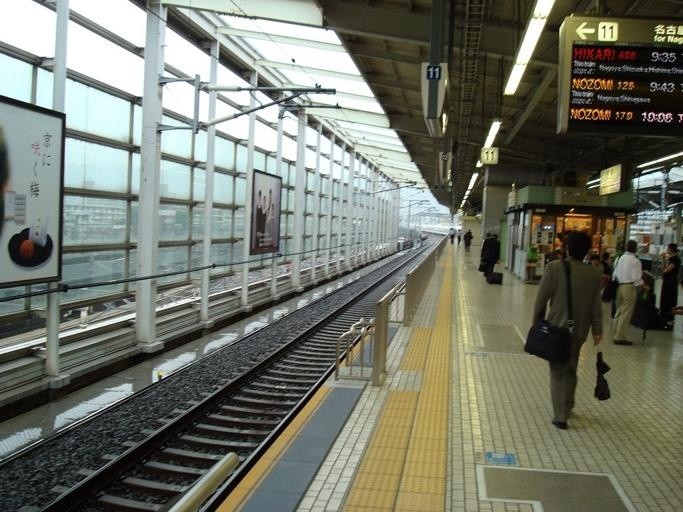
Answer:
[614, 339, 632, 345]
[552, 420, 567, 429]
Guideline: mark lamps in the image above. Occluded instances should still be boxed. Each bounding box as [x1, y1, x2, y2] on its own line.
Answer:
[472, 81, 505, 168]
[503, 0, 554, 97]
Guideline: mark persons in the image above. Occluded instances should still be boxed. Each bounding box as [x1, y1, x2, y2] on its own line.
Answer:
[480, 231, 492, 277]
[482, 234, 501, 283]
[601, 252, 614, 302]
[253, 187, 277, 247]
[590, 255, 600, 268]
[449, 227, 473, 253]
[531, 230, 604, 430]
[582, 250, 592, 265]
[607, 246, 624, 319]
[670, 306, 683, 316]
[657, 242, 682, 322]
[611, 240, 651, 345]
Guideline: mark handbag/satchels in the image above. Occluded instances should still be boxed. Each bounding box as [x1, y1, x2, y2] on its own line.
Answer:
[524, 319, 572, 362]
[487, 273, 502, 284]
[602, 280, 618, 303]
[631, 289, 674, 330]
[478, 264, 488, 272]
